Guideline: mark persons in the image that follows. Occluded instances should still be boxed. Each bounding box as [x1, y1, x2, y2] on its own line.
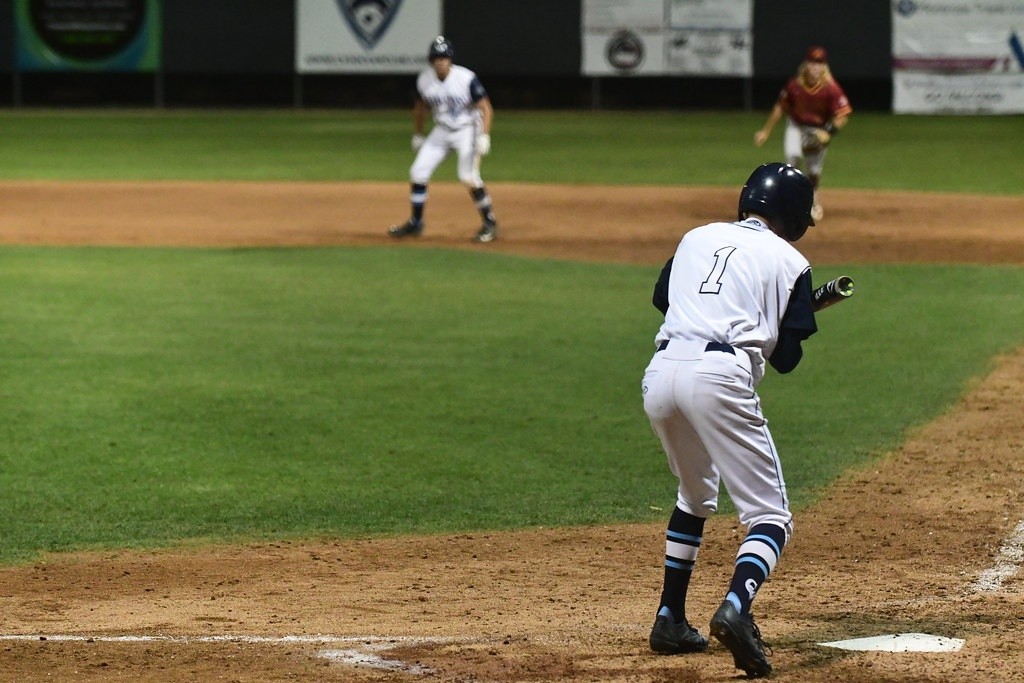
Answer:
[387, 34, 497, 244]
[641, 162, 819, 678]
[753, 45, 851, 223]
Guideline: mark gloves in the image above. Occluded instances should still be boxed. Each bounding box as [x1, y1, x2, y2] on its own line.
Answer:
[474, 134, 491, 155]
[412, 134, 425, 150]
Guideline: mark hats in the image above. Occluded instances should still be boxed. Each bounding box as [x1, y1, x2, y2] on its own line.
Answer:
[428, 36, 454, 62]
[806, 45, 827, 62]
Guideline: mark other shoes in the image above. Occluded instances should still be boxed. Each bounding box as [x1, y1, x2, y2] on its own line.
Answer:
[386, 220, 424, 239]
[474, 226, 498, 243]
[811, 202, 823, 221]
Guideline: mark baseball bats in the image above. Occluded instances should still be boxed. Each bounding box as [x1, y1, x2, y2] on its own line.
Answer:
[811, 275, 856, 312]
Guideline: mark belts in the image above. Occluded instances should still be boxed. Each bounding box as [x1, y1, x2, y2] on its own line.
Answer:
[656, 340, 736, 355]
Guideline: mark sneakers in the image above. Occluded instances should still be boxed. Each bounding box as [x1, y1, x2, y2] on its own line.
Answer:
[649, 615, 709, 655]
[709, 599, 774, 678]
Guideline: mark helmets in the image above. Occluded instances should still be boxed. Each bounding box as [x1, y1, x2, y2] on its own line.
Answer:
[739, 162, 815, 241]
